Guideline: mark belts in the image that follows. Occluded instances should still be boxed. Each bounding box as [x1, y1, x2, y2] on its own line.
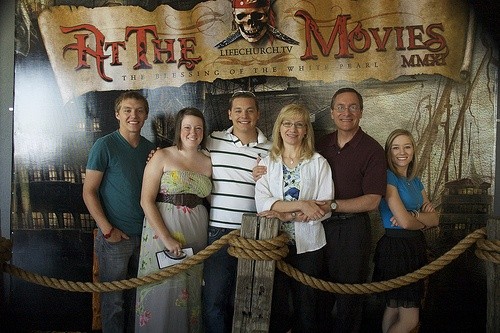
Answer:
[327, 215, 354, 222]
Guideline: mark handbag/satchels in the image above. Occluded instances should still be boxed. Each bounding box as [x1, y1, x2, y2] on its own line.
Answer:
[156, 247, 194, 269]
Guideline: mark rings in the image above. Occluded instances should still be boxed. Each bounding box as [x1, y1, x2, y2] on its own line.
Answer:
[178, 249, 182, 252]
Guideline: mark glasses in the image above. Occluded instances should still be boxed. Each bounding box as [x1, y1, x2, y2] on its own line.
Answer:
[281, 122, 308, 127]
[333, 105, 361, 112]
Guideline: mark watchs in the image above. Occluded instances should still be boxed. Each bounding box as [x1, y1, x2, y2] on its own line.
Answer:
[290, 212, 296, 220]
[330, 200, 338, 212]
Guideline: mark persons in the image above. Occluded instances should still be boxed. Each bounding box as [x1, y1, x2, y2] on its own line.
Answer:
[372, 129, 440, 333]
[254, 104, 334, 333]
[82, 90, 160, 333]
[252, 88, 387, 333]
[145, 90, 273, 332]
[135, 107, 212, 333]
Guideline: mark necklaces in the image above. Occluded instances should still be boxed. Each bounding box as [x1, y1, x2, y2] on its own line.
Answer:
[285, 154, 297, 165]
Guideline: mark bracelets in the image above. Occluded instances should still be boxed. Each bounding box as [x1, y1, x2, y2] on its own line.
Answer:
[102, 227, 114, 239]
[422, 224, 426, 230]
[409, 209, 419, 218]
